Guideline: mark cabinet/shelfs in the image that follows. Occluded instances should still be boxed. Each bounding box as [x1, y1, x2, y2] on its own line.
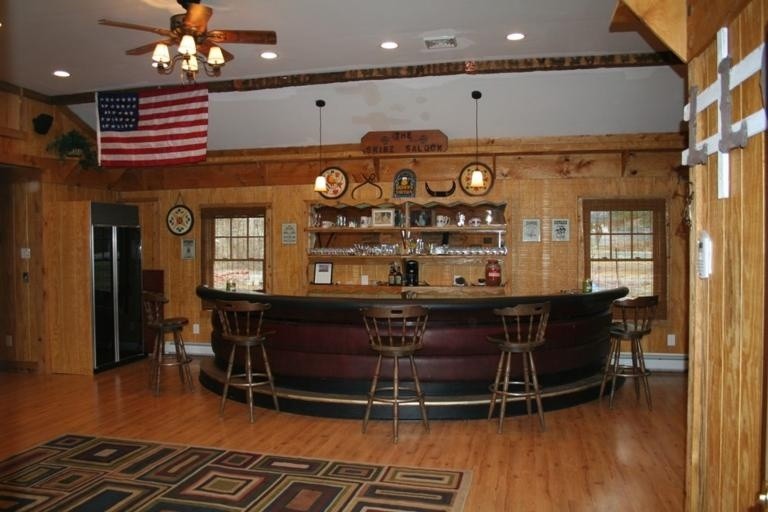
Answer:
[304, 200, 508, 293]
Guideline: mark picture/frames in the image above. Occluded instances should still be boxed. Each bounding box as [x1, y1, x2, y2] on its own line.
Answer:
[372, 208, 396, 227]
[552, 219, 571, 242]
[522, 219, 541, 242]
[181, 239, 196, 259]
[314, 262, 333, 284]
[281, 223, 298, 244]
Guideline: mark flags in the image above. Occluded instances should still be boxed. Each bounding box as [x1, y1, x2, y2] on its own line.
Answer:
[95, 84, 214, 167]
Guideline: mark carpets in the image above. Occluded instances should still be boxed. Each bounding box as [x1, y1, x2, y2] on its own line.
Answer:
[0, 433, 474, 512]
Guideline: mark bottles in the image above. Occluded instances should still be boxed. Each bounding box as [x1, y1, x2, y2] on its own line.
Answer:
[406, 259, 419, 287]
[388, 265, 402, 286]
[484, 259, 502, 287]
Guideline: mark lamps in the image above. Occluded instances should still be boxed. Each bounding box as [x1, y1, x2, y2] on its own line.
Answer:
[314, 99, 327, 192]
[152, 35, 226, 86]
[470, 90, 484, 187]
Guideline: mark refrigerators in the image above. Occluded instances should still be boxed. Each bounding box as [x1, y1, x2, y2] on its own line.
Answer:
[90, 199, 146, 374]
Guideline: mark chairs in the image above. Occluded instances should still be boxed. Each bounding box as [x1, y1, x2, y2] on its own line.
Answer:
[358, 304, 434, 444]
[486, 300, 552, 434]
[214, 299, 280, 424]
[598, 295, 659, 410]
[141, 290, 195, 395]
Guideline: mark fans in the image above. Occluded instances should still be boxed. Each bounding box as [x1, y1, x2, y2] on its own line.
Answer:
[98, 0, 276, 63]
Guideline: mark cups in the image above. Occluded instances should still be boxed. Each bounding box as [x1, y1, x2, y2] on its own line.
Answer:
[435, 209, 496, 227]
[306, 239, 400, 256]
[582, 279, 593, 293]
[226, 279, 236, 291]
[312, 212, 372, 229]
[408, 238, 508, 256]
